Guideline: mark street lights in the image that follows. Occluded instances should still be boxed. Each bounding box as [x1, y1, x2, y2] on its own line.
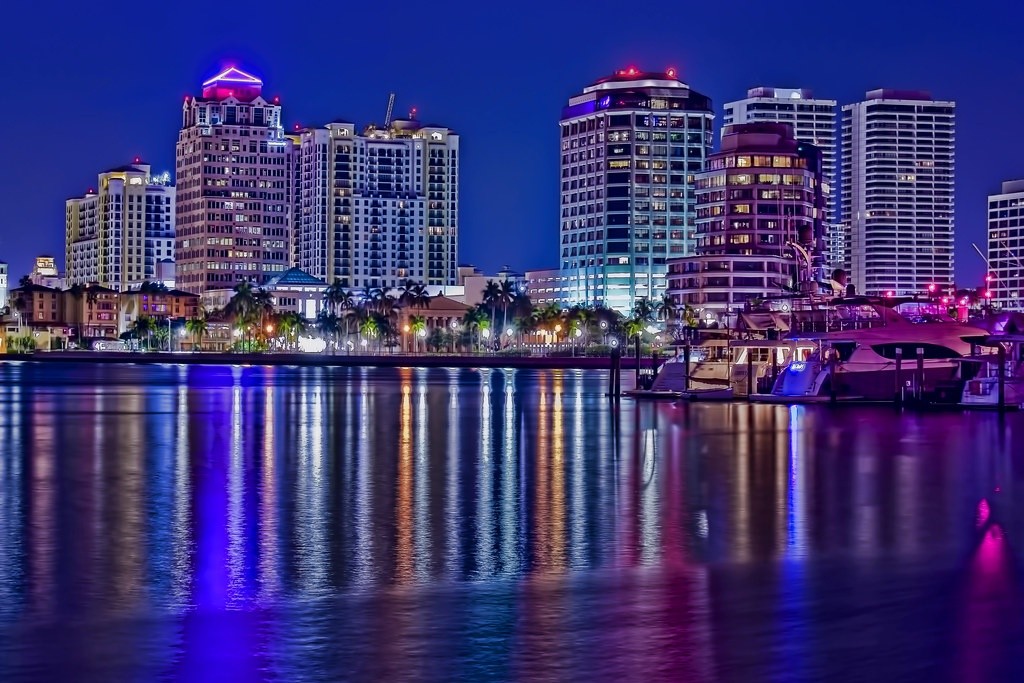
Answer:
[404, 325, 410, 355]
[266, 326, 273, 352]
[247, 326, 251, 352]
[165, 318, 171, 352]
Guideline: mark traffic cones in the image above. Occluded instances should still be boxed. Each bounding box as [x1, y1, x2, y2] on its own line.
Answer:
[14, 312, 20, 354]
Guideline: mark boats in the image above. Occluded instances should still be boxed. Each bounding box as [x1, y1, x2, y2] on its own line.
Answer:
[639, 292, 1024, 409]
